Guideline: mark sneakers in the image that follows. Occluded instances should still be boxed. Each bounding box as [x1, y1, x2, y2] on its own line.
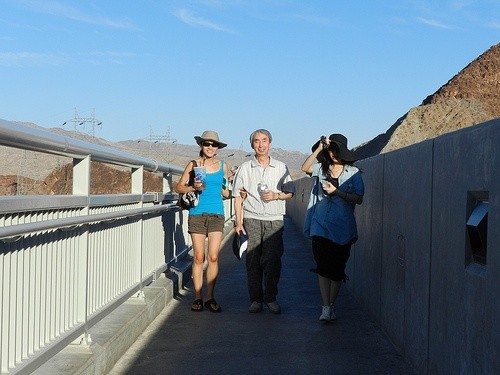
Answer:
[319, 304, 336, 322]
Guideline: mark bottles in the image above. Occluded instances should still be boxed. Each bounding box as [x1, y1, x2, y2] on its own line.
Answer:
[261, 182, 270, 203]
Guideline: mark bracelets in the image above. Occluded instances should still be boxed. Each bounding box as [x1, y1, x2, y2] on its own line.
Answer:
[344, 192, 347, 200]
[276, 192, 280, 200]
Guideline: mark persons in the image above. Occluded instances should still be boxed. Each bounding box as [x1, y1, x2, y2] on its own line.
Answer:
[233, 128, 296, 314]
[301, 133, 365, 322]
[175, 130, 229, 312]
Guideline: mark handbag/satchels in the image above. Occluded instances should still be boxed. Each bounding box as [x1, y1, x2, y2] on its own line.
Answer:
[176, 160, 200, 208]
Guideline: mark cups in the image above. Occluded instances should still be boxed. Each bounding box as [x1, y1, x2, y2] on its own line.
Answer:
[193, 165, 207, 191]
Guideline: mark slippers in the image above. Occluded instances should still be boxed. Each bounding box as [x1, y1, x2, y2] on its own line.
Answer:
[192, 298, 203, 311]
[205, 298, 222, 313]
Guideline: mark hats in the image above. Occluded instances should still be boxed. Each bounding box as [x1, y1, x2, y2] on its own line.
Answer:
[312, 134, 357, 162]
[194, 130, 227, 149]
[233, 230, 248, 260]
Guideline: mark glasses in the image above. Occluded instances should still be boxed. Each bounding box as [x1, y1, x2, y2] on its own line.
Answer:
[200, 142, 219, 147]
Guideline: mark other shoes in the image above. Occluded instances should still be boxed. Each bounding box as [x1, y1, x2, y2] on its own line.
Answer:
[249, 301, 263, 313]
[265, 302, 282, 313]
